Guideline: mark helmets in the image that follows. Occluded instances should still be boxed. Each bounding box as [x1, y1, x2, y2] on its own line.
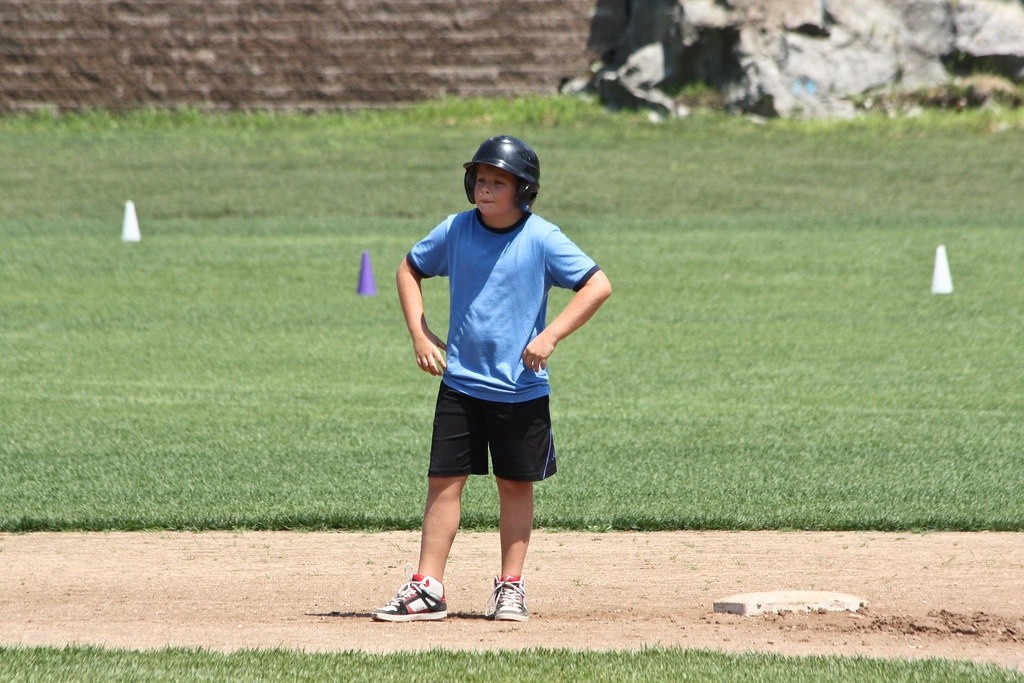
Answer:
[463, 136, 540, 212]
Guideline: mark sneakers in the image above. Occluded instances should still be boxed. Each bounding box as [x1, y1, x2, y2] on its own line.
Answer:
[485, 575, 528, 620]
[372, 563, 448, 620]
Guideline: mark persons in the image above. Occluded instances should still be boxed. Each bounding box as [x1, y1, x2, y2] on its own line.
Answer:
[372, 133, 613, 624]
